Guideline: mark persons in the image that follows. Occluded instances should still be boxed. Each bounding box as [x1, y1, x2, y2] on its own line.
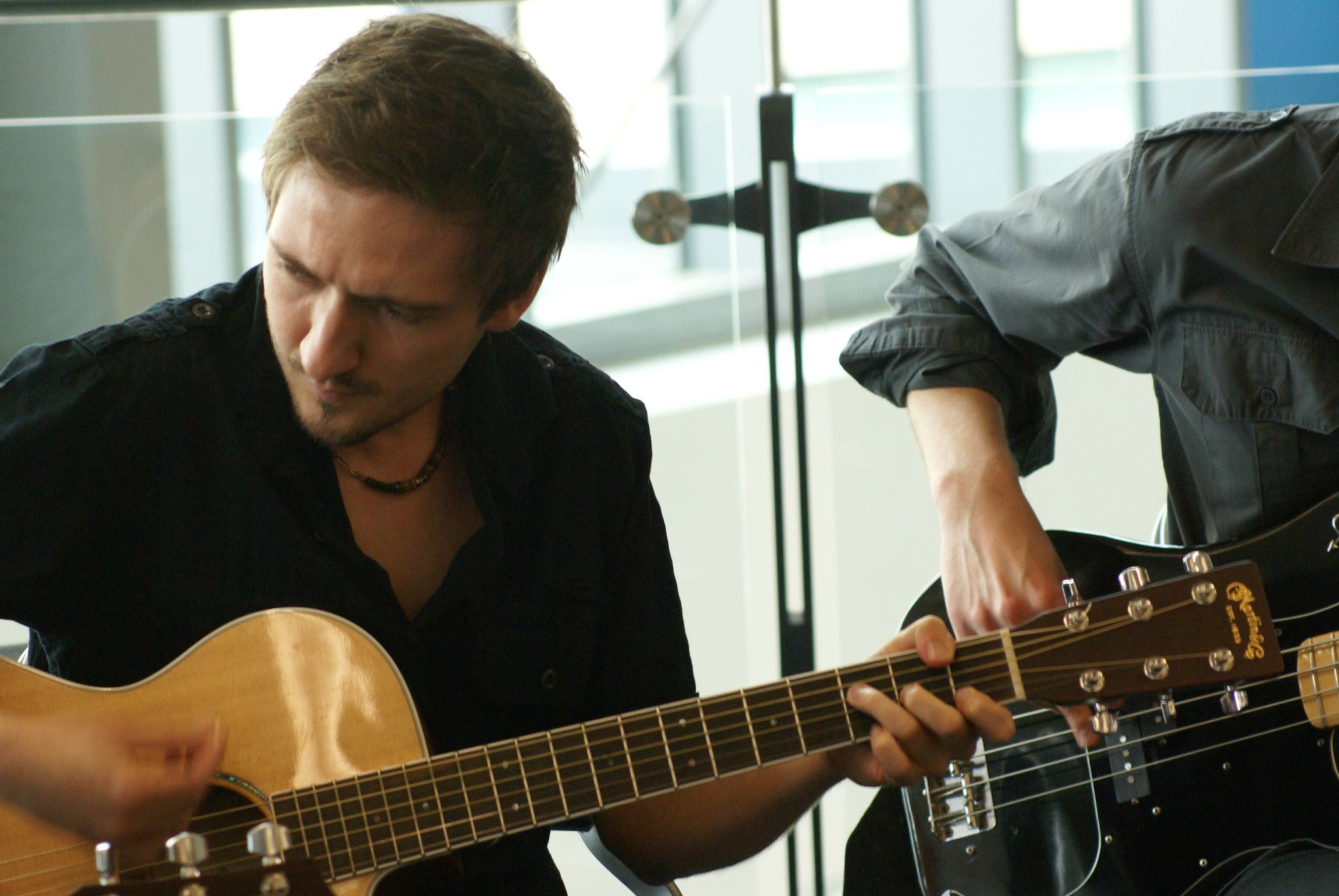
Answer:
[0, 16, 1014, 896]
[838, 101, 1339, 895]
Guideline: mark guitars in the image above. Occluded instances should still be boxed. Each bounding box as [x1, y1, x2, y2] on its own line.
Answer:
[836, 492, 1337, 892]
[0, 547, 1286, 894]
